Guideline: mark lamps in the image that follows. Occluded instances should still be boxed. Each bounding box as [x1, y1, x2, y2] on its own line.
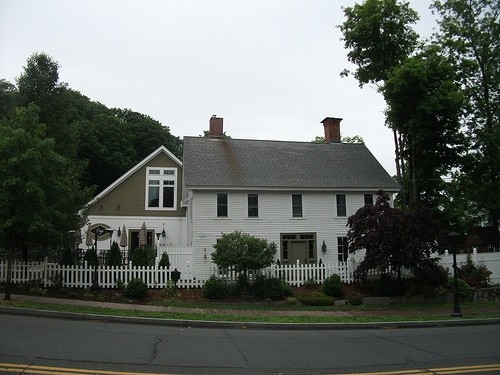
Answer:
[117, 227, 121, 236]
[162, 230, 166, 237]
[322, 241, 327, 253]
[273, 246, 276, 254]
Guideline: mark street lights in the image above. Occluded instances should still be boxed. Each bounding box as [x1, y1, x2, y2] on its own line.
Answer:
[447, 226, 463, 317]
